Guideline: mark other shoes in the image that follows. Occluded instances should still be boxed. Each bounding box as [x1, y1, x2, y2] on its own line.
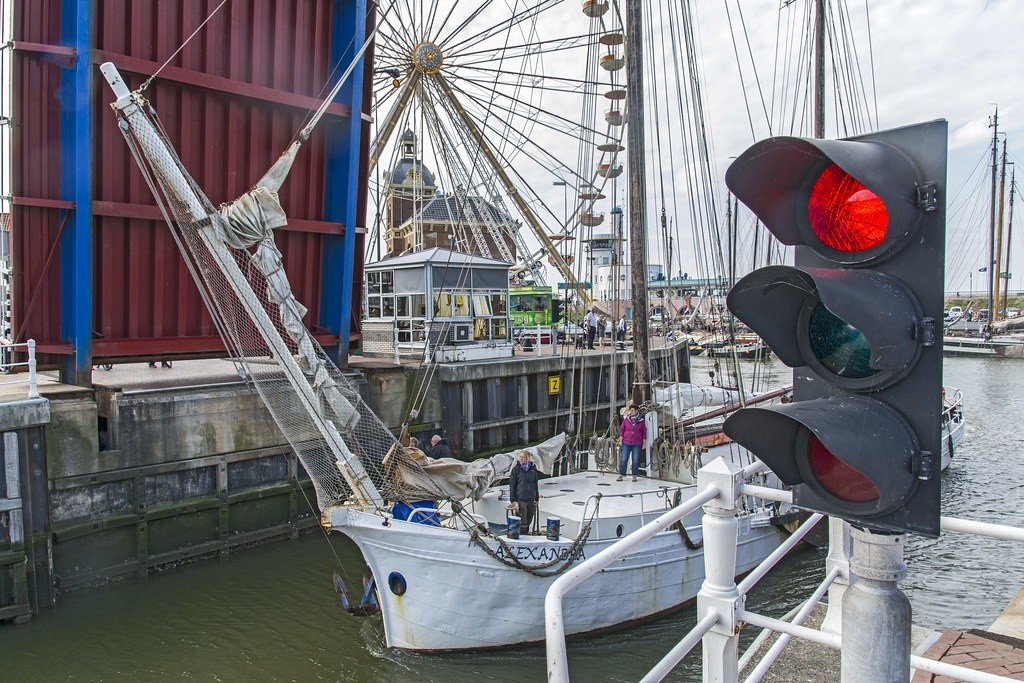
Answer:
[616, 475, 623, 481]
[632, 475, 637, 482]
[581, 343, 627, 351]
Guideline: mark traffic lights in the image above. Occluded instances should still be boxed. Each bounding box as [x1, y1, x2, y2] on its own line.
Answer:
[551, 299, 564, 322]
[725, 117, 949, 542]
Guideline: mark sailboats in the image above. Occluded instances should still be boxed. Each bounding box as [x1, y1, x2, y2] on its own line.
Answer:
[639, 104, 1023, 360]
[95, 0, 965, 652]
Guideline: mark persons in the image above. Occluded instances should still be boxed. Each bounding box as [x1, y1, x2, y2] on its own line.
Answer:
[597, 314, 606, 347]
[509, 450, 540, 537]
[582, 310, 591, 350]
[586, 306, 598, 350]
[617, 404, 647, 482]
[618, 314, 627, 350]
[428, 435, 453, 459]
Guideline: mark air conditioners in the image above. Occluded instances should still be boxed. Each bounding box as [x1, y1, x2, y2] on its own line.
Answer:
[447, 322, 475, 343]
